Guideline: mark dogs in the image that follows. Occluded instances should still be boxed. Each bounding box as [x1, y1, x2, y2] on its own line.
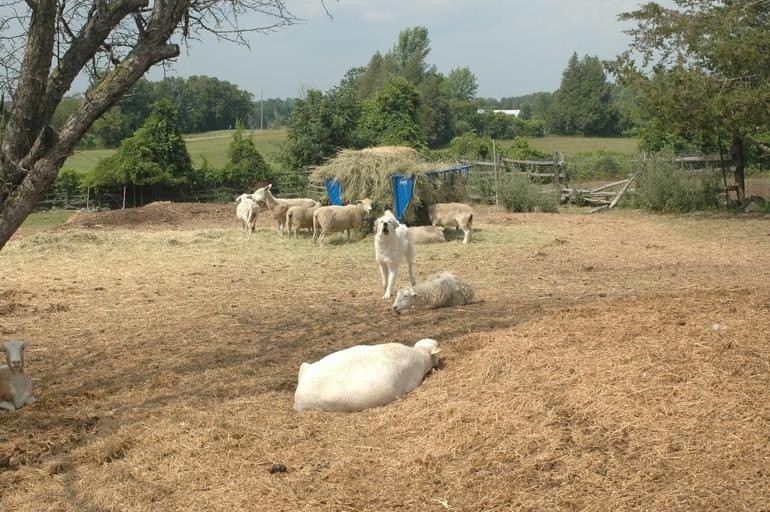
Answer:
[371, 215, 414, 301]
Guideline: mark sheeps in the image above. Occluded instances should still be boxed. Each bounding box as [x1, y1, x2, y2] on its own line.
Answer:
[0, 337, 39, 413]
[292, 337, 445, 413]
[408, 225, 448, 244]
[390, 271, 484, 317]
[283, 204, 315, 239]
[250, 183, 317, 235]
[307, 198, 373, 248]
[234, 192, 262, 234]
[416, 198, 474, 244]
